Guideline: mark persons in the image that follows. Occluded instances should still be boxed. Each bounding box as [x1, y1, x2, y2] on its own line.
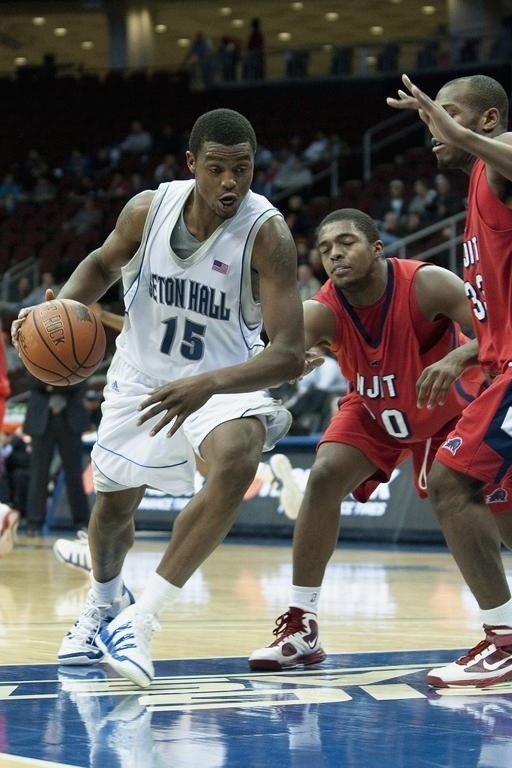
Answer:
[426, 695, 512, 739]
[183, 31, 214, 85]
[1, 373, 91, 540]
[249, 131, 465, 302]
[291, 347, 348, 436]
[386, 69, 510, 692]
[247, 205, 512, 691]
[40, 55, 57, 80]
[0, 122, 195, 314]
[245, 17, 264, 81]
[58, 669, 162, 768]
[10, 105, 305, 692]
[218, 35, 240, 80]
[257, 694, 325, 768]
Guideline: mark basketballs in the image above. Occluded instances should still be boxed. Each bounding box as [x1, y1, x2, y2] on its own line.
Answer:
[18, 298, 106, 386]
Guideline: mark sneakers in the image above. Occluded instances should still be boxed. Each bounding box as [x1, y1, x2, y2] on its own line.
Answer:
[247, 605, 328, 673]
[56, 584, 136, 667]
[53, 538, 94, 576]
[0, 502, 22, 559]
[94, 602, 159, 688]
[424, 623, 511, 691]
